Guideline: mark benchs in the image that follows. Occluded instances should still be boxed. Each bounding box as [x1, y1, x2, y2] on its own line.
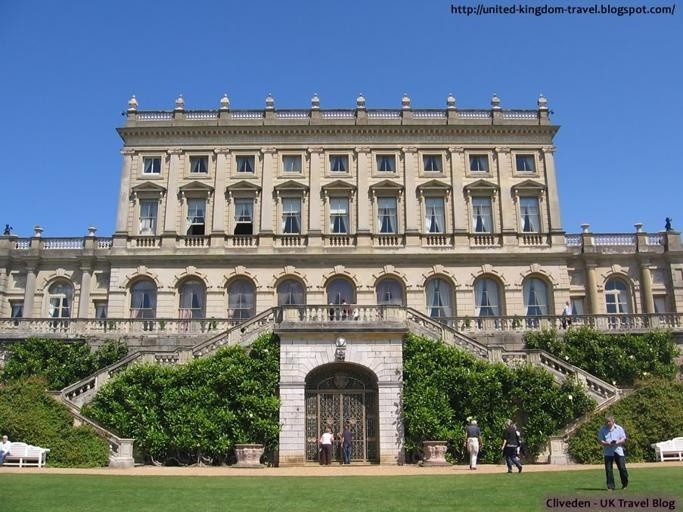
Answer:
[651, 437, 682, 462]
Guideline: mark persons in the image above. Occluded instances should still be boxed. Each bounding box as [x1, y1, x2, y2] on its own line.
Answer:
[501, 419, 522, 473]
[564, 301, 572, 329]
[0, 435, 11, 467]
[464, 420, 483, 470]
[597, 415, 628, 492]
[320, 428, 334, 465]
[339, 425, 353, 464]
